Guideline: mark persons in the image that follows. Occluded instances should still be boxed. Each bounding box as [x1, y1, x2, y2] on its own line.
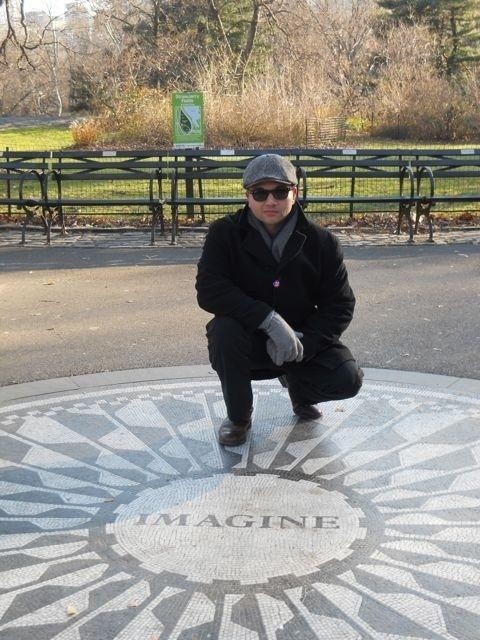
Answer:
[194, 151, 366, 447]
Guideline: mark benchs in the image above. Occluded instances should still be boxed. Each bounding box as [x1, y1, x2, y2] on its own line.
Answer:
[41, 151, 168, 243]
[414, 148, 478, 243]
[0, 152, 49, 244]
[170, 147, 414, 244]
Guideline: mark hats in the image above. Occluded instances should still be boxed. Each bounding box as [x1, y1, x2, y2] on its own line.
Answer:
[242, 154, 298, 187]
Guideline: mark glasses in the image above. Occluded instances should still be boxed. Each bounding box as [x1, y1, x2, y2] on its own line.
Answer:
[247, 185, 296, 202]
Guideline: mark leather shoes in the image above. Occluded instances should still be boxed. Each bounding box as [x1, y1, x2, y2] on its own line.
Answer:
[278, 375, 322, 420]
[218, 412, 252, 446]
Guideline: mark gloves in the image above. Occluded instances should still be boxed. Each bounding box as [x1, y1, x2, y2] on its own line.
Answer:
[259, 310, 305, 366]
